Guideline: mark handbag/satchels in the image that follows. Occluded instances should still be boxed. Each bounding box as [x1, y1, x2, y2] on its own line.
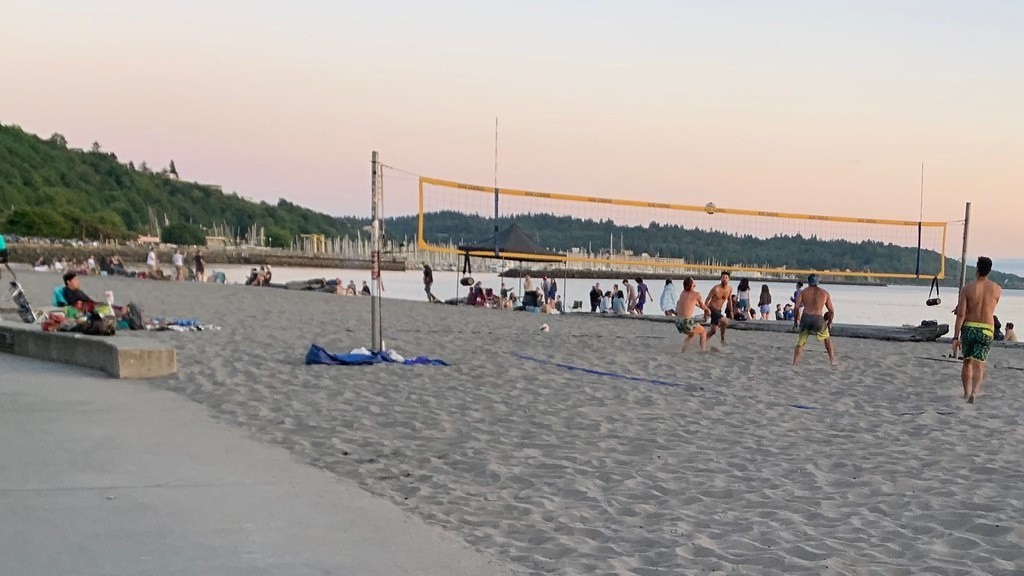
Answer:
[82, 313, 116, 336]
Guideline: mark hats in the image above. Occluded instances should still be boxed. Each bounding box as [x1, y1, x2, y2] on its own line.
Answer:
[808, 274, 819, 285]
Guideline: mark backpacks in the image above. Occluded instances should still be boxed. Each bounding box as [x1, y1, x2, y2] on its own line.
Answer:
[126, 301, 146, 330]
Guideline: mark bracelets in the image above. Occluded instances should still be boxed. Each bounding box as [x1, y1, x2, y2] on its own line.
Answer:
[952, 337, 958, 340]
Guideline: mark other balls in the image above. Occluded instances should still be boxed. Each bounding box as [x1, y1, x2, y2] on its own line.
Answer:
[539, 323, 550, 333]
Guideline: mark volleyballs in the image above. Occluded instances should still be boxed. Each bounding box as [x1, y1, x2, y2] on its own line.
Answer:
[705, 202, 717, 215]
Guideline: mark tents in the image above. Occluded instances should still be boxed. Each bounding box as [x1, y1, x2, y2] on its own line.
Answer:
[457, 222, 566, 313]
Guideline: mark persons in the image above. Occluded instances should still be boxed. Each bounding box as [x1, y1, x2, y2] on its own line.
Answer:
[466, 275, 676, 317]
[704, 271, 734, 346]
[0, 234, 16, 281]
[361, 281, 370, 295]
[33, 255, 127, 276]
[792, 273, 839, 367]
[146, 248, 156, 274]
[63, 272, 126, 315]
[245, 264, 272, 287]
[674, 277, 711, 353]
[192, 249, 205, 281]
[421, 260, 436, 303]
[993, 315, 1018, 342]
[725, 278, 803, 321]
[172, 249, 187, 280]
[346, 280, 356, 295]
[952, 256, 1001, 404]
